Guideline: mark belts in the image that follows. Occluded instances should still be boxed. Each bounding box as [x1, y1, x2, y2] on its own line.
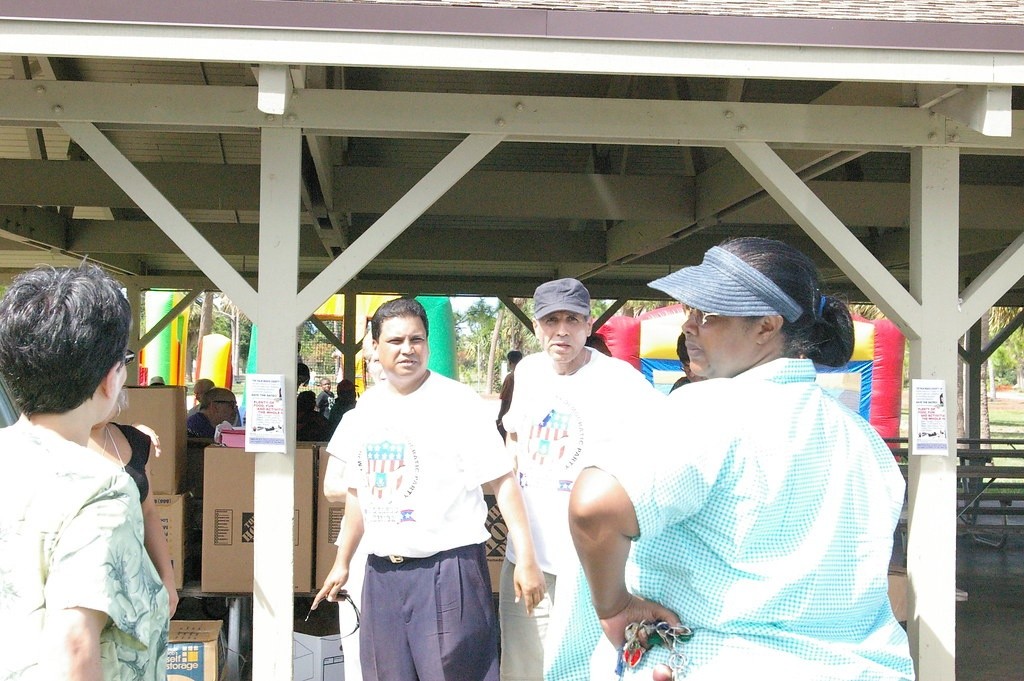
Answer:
[384, 555, 410, 563]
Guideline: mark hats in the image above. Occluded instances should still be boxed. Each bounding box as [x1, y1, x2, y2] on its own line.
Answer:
[533, 278, 590, 320]
[647, 245, 804, 323]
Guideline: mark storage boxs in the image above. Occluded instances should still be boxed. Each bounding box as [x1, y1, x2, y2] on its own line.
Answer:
[99, 386, 507, 681]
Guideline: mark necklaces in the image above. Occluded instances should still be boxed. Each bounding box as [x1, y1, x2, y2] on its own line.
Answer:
[99, 426, 109, 458]
[567, 350, 588, 375]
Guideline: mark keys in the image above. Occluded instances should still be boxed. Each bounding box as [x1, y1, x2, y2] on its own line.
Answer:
[623, 621, 672, 665]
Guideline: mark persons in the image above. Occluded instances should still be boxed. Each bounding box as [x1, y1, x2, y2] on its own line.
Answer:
[499, 277, 670, 681]
[584, 333, 612, 357]
[671, 332, 707, 392]
[86, 392, 179, 620]
[548, 237, 915, 681]
[296, 363, 357, 442]
[496, 351, 523, 442]
[0, 263, 167, 681]
[150, 375, 241, 502]
[310, 297, 548, 681]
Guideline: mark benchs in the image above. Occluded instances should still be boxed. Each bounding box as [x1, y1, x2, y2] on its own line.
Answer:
[880, 437, 1024, 547]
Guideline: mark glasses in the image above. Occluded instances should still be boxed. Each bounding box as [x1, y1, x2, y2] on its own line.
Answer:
[208, 401, 236, 409]
[682, 303, 716, 328]
[680, 362, 691, 372]
[124, 349, 135, 365]
